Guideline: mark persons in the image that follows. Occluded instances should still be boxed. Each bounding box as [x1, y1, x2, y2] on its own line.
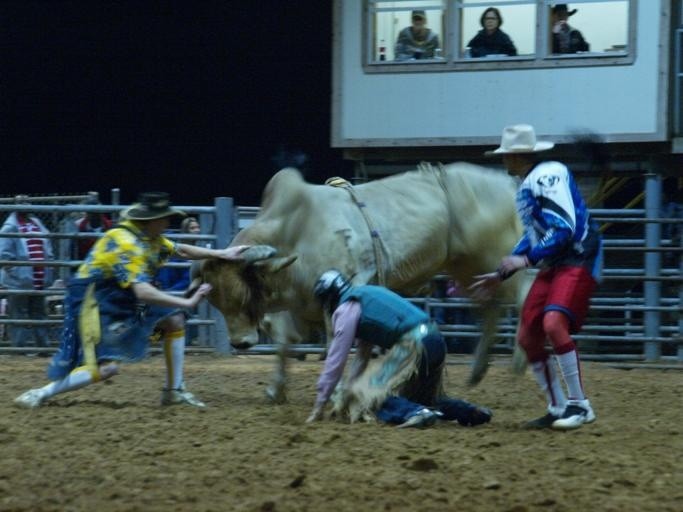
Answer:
[304, 266, 493, 429]
[466, 7, 518, 57]
[155, 217, 200, 345]
[394, 10, 438, 61]
[0, 193, 55, 357]
[551, 3, 589, 54]
[466, 123, 604, 429]
[58, 196, 112, 280]
[12, 192, 250, 407]
[415, 279, 463, 353]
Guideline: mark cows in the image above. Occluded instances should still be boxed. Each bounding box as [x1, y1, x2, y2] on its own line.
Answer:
[184, 160, 544, 422]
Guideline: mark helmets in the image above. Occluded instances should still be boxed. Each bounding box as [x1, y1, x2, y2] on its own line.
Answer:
[312, 270, 348, 319]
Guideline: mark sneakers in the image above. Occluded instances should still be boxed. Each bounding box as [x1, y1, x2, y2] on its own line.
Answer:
[524, 400, 595, 430]
[394, 409, 439, 428]
[13, 388, 41, 411]
[466, 408, 491, 426]
[162, 388, 206, 408]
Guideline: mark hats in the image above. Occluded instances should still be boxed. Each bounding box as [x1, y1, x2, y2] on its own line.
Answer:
[412, 10, 425, 19]
[548, 4, 576, 16]
[485, 125, 555, 156]
[120, 192, 186, 221]
